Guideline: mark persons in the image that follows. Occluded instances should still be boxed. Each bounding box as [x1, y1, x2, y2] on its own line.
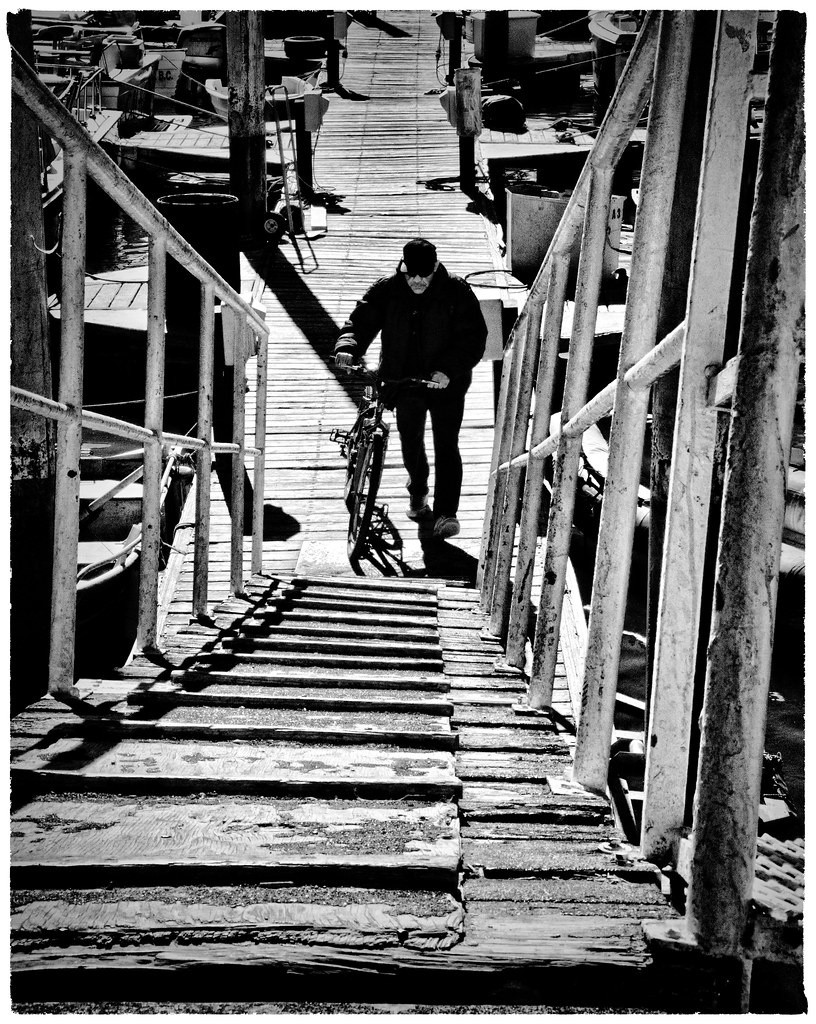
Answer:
[335, 235, 490, 539]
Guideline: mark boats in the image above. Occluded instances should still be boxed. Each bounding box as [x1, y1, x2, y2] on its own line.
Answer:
[31, 9, 231, 116]
[548, 409, 806, 587]
[73, 440, 186, 593]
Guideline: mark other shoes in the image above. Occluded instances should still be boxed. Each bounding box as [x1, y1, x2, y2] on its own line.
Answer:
[432, 516, 461, 540]
[407, 486, 429, 518]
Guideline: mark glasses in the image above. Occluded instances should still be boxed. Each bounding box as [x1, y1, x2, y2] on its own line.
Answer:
[406, 268, 433, 278]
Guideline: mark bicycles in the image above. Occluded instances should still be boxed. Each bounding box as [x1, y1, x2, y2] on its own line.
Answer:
[330, 355, 440, 563]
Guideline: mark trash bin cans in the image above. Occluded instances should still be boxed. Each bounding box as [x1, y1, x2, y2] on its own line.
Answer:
[507, 185, 569, 275]
[155, 193, 240, 334]
